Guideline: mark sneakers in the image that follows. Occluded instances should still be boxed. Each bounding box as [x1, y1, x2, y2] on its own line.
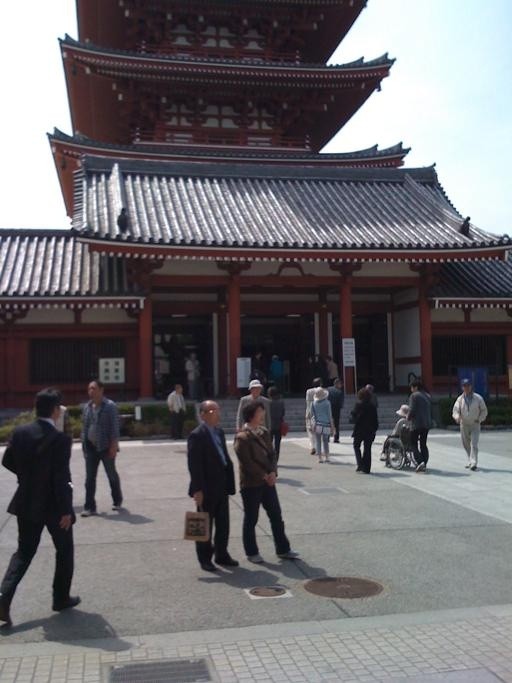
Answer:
[247, 553, 265, 564]
[422, 467, 426, 471]
[415, 462, 425, 473]
[277, 550, 301, 558]
[81, 508, 97, 518]
[112, 504, 121, 510]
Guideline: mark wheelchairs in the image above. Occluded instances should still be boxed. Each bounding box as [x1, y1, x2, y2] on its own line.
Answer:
[380, 420, 429, 470]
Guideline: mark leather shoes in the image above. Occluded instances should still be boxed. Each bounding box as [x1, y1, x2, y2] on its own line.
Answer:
[0, 613, 13, 630]
[52, 594, 81, 613]
[213, 556, 239, 567]
[200, 560, 219, 572]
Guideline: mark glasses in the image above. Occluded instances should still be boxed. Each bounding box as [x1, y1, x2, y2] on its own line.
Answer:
[202, 409, 221, 415]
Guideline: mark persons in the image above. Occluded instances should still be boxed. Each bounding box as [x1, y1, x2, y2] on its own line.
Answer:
[452, 379, 487, 470]
[236, 380, 270, 430]
[310, 387, 335, 463]
[2, 389, 81, 626]
[305, 355, 337, 387]
[167, 384, 186, 440]
[349, 384, 377, 474]
[328, 379, 343, 442]
[251, 351, 282, 396]
[381, 371, 431, 473]
[233, 402, 299, 562]
[185, 353, 200, 399]
[267, 386, 285, 462]
[306, 378, 321, 454]
[80, 378, 123, 516]
[188, 400, 239, 569]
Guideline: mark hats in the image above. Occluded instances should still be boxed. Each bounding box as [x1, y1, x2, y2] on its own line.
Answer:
[248, 380, 263, 391]
[460, 378, 473, 386]
[395, 404, 410, 417]
[313, 387, 329, 401]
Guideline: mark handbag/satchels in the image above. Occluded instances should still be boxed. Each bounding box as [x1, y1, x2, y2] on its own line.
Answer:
[279, 418, 289, 436]
[183, 501, 211, 543]
